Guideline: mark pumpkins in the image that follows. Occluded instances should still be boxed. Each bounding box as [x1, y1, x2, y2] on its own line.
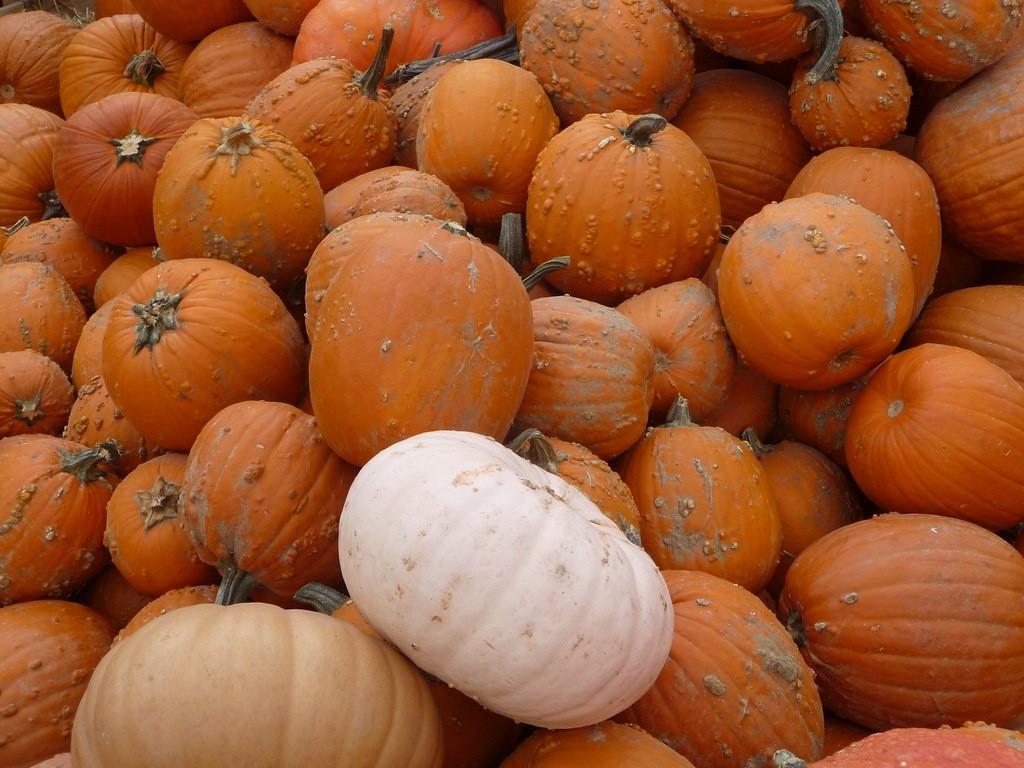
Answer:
[0, 0, 1024, 768]
